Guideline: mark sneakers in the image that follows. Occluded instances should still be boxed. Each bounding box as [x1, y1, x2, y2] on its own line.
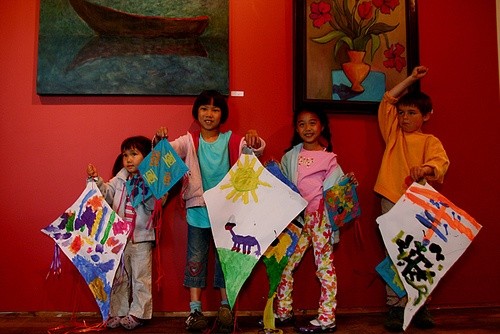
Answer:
[184, 309, 208, 330]
[385, 307, 404, 331]
[217, 304, 232, 332]
[413, 304, 436, 330]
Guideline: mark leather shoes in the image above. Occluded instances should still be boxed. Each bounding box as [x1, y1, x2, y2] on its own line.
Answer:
[258, 312, 297, 327]
[296, 317, 337, 334]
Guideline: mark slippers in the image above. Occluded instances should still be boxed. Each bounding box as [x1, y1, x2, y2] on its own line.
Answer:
[107, 317, 121, 328]
[120, 314, 140, 330]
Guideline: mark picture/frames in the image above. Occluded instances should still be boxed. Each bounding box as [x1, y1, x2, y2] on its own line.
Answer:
[292, 0, 422, 116]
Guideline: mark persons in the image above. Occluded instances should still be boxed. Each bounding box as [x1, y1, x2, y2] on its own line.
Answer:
[152, 90, 266, 332]
[264, 103, 359, 334]
[88, 136, 168, 329]
[373, 65, 451, 332]
